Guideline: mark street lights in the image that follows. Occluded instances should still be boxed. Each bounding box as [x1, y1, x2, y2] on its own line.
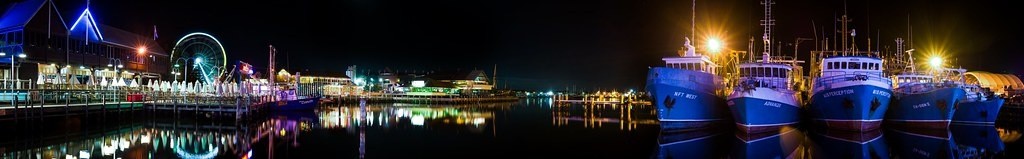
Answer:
[107, 57, 123, 104]
[170, 67, 180, 81]
[0, 43, 28, 107]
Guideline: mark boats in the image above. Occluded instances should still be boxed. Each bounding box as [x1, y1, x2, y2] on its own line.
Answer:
[644, 0, 1006, 130]
